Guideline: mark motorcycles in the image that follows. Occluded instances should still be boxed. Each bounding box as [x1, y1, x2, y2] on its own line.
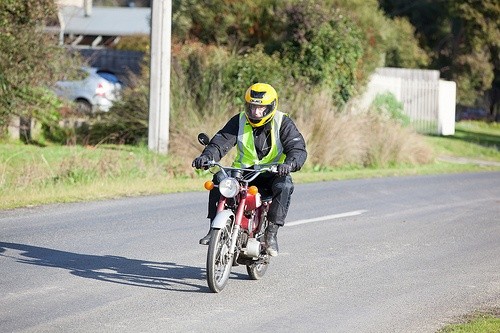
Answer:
[185, 133, 283, 293]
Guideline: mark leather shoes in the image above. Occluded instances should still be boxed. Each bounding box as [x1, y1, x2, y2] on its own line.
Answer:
[264, 224, 280, 256]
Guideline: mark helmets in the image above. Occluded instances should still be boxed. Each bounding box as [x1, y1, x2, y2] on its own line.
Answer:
[244, 83, 278, 127]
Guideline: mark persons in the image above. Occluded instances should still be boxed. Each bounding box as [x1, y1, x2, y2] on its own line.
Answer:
[191, 82, 308, 257]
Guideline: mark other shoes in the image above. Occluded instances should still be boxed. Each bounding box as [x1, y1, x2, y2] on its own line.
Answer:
[199, 224, 214, 245]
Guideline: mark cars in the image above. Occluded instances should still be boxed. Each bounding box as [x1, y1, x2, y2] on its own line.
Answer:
[39, 65, 124, 114]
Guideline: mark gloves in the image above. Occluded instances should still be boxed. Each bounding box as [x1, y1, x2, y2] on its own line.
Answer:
[191, 154, 210, 170]
[276, 163, 291, 177]
